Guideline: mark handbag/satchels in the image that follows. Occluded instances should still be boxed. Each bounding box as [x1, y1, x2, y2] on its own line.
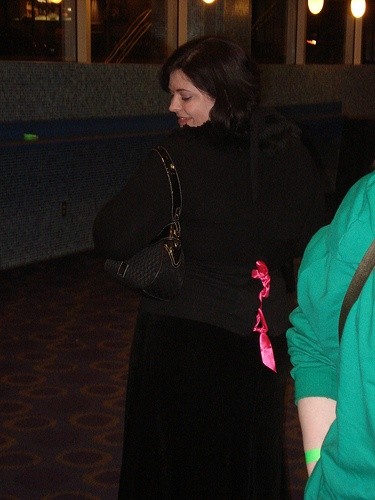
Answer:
[96, 147, 183, 302]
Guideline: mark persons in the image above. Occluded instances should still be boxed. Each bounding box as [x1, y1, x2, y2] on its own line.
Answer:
[94, 36, 313, 500]
[287, 167, 375, 500]
